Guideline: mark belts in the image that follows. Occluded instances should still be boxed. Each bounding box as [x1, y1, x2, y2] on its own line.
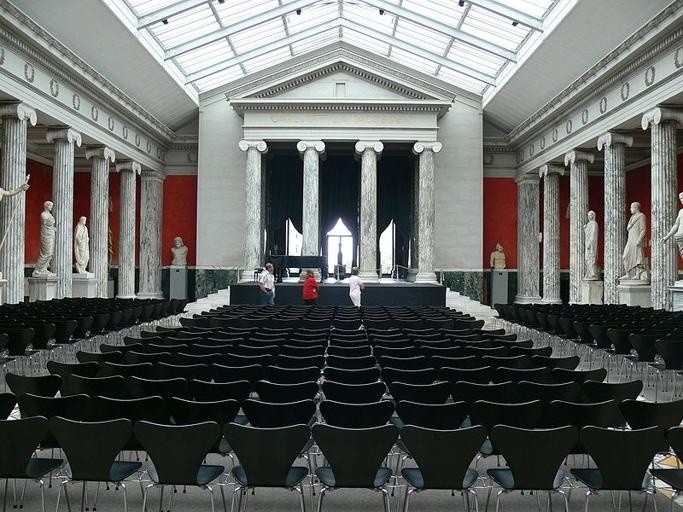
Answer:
[266, 289, 272, 291]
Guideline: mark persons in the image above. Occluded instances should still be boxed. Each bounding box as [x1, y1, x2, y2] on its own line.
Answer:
[74, 216, 90, 274]
[169, 237, 190, 265]
[584, 211, 601, 279]
[348, 266, 364, 307]
[32, 201, 57, 277]
[616, 201, 653, 282]
[0, 173, 29, 283]
[659, 192, 683, 265]
[490, 243, 511, 269]
[259, 263, 275, 305]
[303, 271, 321, 306]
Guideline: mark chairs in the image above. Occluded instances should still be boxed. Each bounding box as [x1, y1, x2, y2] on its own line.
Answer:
[372, 339, 411, 347]
[255, 380, 320, 402]
[507, 345, 553, 357]
[0, 416, 72, 512]
[472, 328, 505, 336]
[494, 339, 534, 348]
[50, 416, 147, 512]
[482, 333, 518, 343]
[407, 333, 441, 345]
[557, 302, 571, 357]
[291, 332, 328, 340]
[324, 367, 380, 384]
[368, 328, 399, 335]
[382, 366, 437, 386]
[508, 303, 532, 340]
[439, 328, 469, 337]
[388, 381, 455, 430]
[0, 303, 19, 396]
[167, 336, 203, 345]
[369, 333, 403, 342]
[277, 354, 325, 369]
[571, 304, 590, 371]
[208, 337, 244, 345]
[76, 350, 123, 365]
[643, 427, 683, 512]
[126, 351, 172, 366]
[289, 338, 328, 348]
[155, 326, 190, 332]
[491, 304, 507, 334]
[21, 392, 90, 488]
[238, 344, 279, 355]
[425, 319, 453, 329]
[216, 331, 250, 339]
[6, 373, 62, 397]
[391, 400, 469, 496]
[517, 381, 575, 428]
[208, 364, 264, 387]
[262, 327, 293, 333]
[283, 344, 324, 355]
[321, 381, 386, 404]
[319, 400, 395, 497]
[440, 366, 493, 386]
[329, 339, 369, 346]
[328, 346, 372, 357]
[158, 361, 207, 382]
[414, 339, 452, 347]
[333, 319, 361, 330]
[629, 305, 683, 402]
[455, 339, 491, 347]
[620, 399, 673, 430]
[116, 298, 135, 347]
[532, 355, 580, 371]
[273, 318, 300, 329]
[135, 298, 154, 337]
[154, 298, 188, 329]
[471, 400, 542, 496]
[330, 329, 365, 334]
[265, 366, 320, 385]
[226, 352, 272, 367]
[241, 399, 317, 496]
[141, 331, 176, 339]
[583, 380, 644, 430]
[147, 343, 190, 354]
[380, 355, 427, 370]
[590, 303, 628, 383]
[374, 345, 416, 357]
[124, 336, 163, 347]
[19, 299, 54, 376]
[482, 354, 530, 376]
[531, 303, 557, 346]
[444, 333, 479, 342]
[212, 317, 239, 328]
[552, 367, 607, 393]
[95, 395, 164, 490]
[395, 319, 424, 330]
[496, 366, 548, 384]
[311, 423, 401, 512]
[193, 304, 476, 321]
[455, 381, 514, 416]
[227, 327, 260, 333]
[465, 346, 506, 357]
[330, 333, 366, 340]
[455, 319, 484, 330]
[364, 318, 392, 329]
[254, 332, 289, 340]
[0, 393, 16, 419]
[297, 327, 330, 334]
[100, 342, 144, 354]
[192, 379, 251, 402]
[134, 420, 227, 512]
[431, 355, 477, 374]
[179, 331, 212, 339]
[303, 318, 331, 330]
[67, 373, 125, 396]
[179, 317, 209, 328]
[171, 396, 241, 491]
[47, 361, 100, 378]
[396, 425, 487, 512]
[223, 423, 311, 512]
[421, 346, 461, 356]
[248, 338, 285, 346]
[53, 298, 79, 364]
[326, 355, 377, 369]
[193, 326, 223, 333]
[193, 343, 233, 356]
[549, 400, 618, 468]
[105, 361, 154, 381]
[179, 351, 224, 369]
[126, 376, 188, 403]
[485, 425, 578, 512]
[241, 318, 269, 328]
[79, 297, 116, 354]
[403, 328, 434, 336]
[567, 426, 666, 512]
